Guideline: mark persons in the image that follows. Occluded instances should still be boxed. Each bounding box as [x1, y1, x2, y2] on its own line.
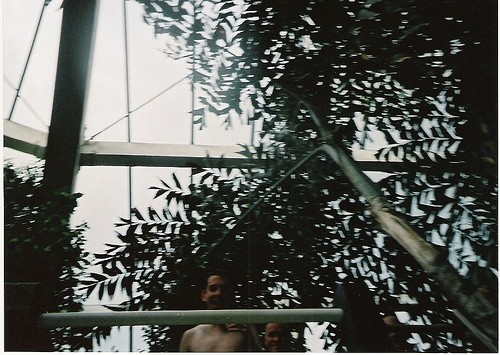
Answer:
[179, 270, 252, 353]
[265, 321, 292, 352]
